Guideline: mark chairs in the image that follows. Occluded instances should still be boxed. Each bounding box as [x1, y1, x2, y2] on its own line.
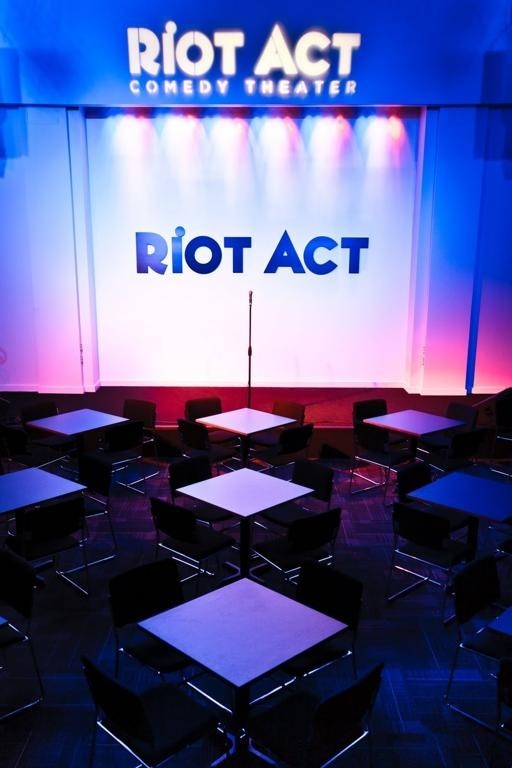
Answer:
[260, 460, 335, 537]
[272, 560, 365, 696]
[122, 399, 156, 436]
[151, 497, 234, 593]
[390, 468, 472, 587]
[108, 555, 191, 691]
[418, 404, 481, 465]
[260, 424, 315, 483]
[248, 661, 387, 768]
[53, 460, 118, 576]
[352, 399, 418, 467]
[84, 420, 145, 496]
[320, 439, 388, 493]
[248, 508, 343, 589]
[444, 557, 510, 731]
[82, 654, 236, 767]
[185, 398, 238, 448]
[168, 457, 239, 530]
[25, 492, 90, 586]
[0, 544, 45, 709]
[253, 402, 306, 449]
[177, 417, 234, 477]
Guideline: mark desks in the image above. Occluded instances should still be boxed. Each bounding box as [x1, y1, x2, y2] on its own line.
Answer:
[362, 409, 466, 469]
[0, 467, 88, 548]
[136, 577, 349, 715]
[176, 468, 315, 585]
[406, 471, 512, 554]
[27, 409, 130, 459]
[197, 407, 296, 475]
